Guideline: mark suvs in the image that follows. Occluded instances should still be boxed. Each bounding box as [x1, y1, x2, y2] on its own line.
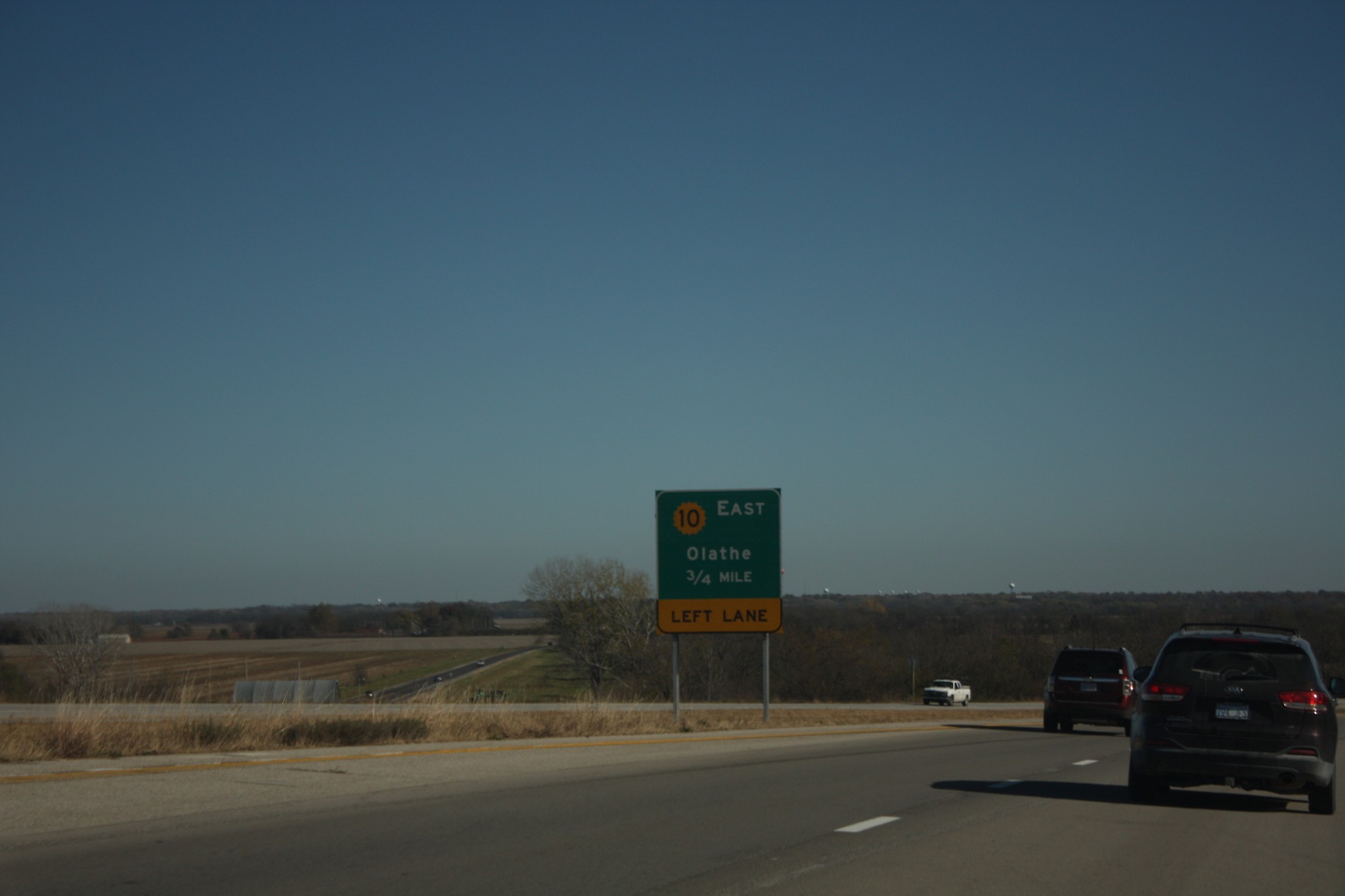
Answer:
[1129, 620, 1344, 814]
[1042, 645, 1139, 735]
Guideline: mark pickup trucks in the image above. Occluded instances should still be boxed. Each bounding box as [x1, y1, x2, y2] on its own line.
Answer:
[922, 679, 971, 706]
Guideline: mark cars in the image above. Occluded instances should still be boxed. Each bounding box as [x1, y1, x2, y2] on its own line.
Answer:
[365, 639, 552, 698]
